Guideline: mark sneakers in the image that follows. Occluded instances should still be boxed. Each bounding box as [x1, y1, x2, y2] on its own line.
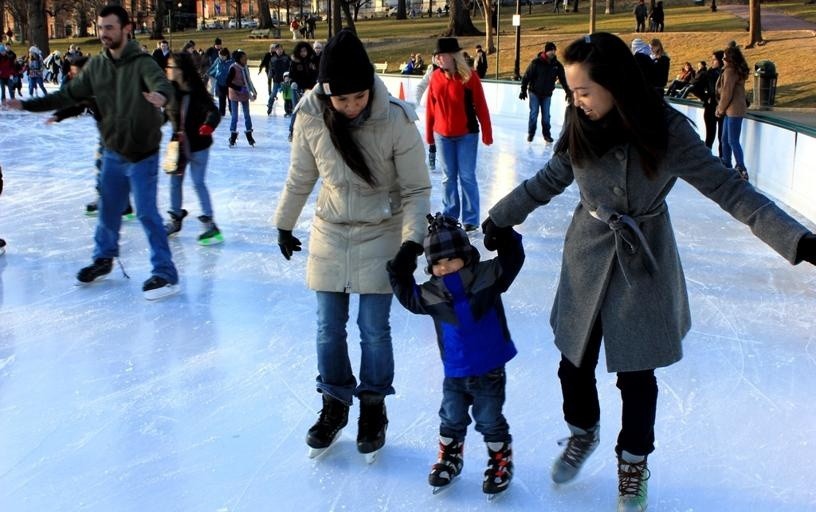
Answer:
[161, 211, 187, 235]
[143, 276, 170, 290]
[77, 259, 112, 282]
[0, 238, 5, 247]
[197, 216, 220, 240]
[528, 135, 534, 141]
[544, 136, 554, 142]
[465, 224, 477, 231]
[551, 420, 600, 483]
[87, 202, 97, 211]
[430, 435, 464, 485]
[428, 143, 436, 152]
[393, 243, 417, 274]
[121, 207, 131, 215]
[483, 442, 513, 494]
[244, 130, 255, 145]
[356, 393, 389, 453]
[617, 451, 651, 512]
[229, 132, 238, 146]
[734, 164, 748, 181]
[305, 394, 349, 448]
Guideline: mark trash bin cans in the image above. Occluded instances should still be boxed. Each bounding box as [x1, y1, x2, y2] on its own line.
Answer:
[274, 29, 280, 38]
[754, 60, 778, 107]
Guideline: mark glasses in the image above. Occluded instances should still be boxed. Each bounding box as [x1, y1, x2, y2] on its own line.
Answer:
[722, 57, 727, 61]
[166, 66, 177, 69]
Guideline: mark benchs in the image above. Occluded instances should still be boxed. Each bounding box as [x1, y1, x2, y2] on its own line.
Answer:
[399, 61, 429, 76]
[369, 61, 388, 74]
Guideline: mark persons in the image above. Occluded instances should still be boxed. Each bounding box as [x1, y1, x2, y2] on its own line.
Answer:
[299, 15, 308, 39]
[258, 43, 278, 96]
[691, 52, 726, 159]
[288, 15, 301, 40]
[631, 35, 656, 89]
[204, 38, 222, 96]
[518, 41, 569, 144]
[306, 13, 318, 40]
[155, 42, 161, 49]
[272, 26, 433, 465]
[482, 31, 816, 508]
[187, 40, 204, 82]
[312, 42, 323, 70]
[634, 1, 648, 33]
[463, 50, 475, 70]
[226, 48, 256, 148]
[666, 60, 695, 101]
[0, 25, 47, 102]
[47, 40, 92, 91]
[289, 43, 317, 91]
[474, 45, 488, 81]
[650, 2, 667, 32]
[277, 72, 295, 118]
[265, 44, 290, 118]
[163, 52, 224, 246]
[650, 36, 671, 100]
[716, 45, 752, 182]
[204, 49, 236, 116]
[647, 3, 656, 33]
[182, 43, 194, 56]
[0, 5, 183, 289]
[413, 52, 440, 170]
[384, 210, 525, 503]
[140, 43, 148, 54]
[41, 98, 132, 217]
[413, 53, 423, 77]
[424, 38, 495, 234]
[677, 68, 686, 80]
[675, 61, 709, 99]
[400, 53, 415, 75]
[152, 40, 171, 67]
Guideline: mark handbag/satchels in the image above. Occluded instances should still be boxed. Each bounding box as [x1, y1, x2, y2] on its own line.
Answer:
[163, 141, 186, 174]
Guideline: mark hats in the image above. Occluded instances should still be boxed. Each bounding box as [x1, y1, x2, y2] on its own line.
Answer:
[313, 40, 322, 49]
[545, 43, 557, 53]
[423, 212, 472, 274]
[435, 39, 463, 55]
[632, 39, 644, 51]
[318, 30, 374, 97]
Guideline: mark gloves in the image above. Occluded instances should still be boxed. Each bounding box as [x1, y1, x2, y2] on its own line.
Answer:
[482, 218, 497, 251]
[565, 94, 571, 102]
[519, 92, 527, 100]
[277, 229, 302, 258]
[199, 125, 212, 135]
[241, 86, 248, 95]
[800, 233, 815, 266]
